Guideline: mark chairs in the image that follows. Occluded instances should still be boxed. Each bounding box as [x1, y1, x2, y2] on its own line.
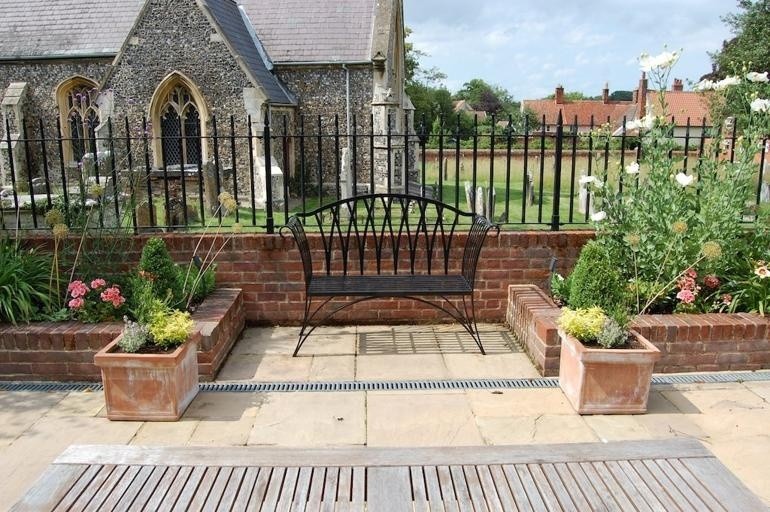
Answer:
[276, 192, 500, 357]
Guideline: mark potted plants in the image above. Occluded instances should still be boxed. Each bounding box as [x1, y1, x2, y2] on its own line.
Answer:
[93, 276, 203, 423]
[555, 305, 662, 415]
